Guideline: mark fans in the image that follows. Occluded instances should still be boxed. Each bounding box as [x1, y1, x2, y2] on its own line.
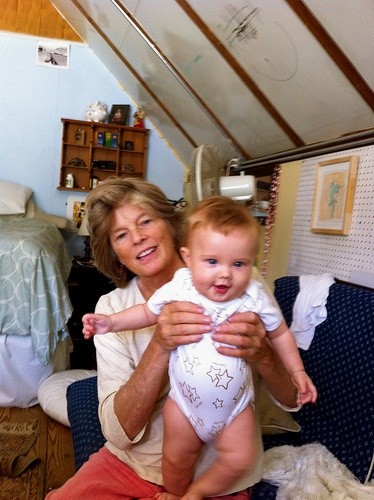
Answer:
[188, 142, 256, 208]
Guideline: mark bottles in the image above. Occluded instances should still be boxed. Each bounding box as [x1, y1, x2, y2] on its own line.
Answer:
[65, 173, 74, 188]
[92, 176, 98, 189]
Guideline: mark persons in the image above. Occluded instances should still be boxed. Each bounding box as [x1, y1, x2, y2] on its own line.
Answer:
[43, 173, 303, 499]
[82, 194, 317, 500]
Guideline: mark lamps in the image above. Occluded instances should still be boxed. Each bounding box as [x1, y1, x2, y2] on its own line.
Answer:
[77, 215, 93, 262]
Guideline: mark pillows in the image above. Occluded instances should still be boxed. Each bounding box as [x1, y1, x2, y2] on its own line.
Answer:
[0, 179, 33, 215]
[253, 376, 301, 435]
[36, 369, 98, 427]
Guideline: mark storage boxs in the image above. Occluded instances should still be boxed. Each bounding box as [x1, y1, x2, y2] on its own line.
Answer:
[93, 130, 118, 148]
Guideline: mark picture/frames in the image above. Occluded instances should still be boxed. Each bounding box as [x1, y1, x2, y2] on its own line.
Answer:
[66, 195, 86, 232]
[107, 104, 130, 125]
[310, 155, 359, 235]
[35, 41, 70, 70]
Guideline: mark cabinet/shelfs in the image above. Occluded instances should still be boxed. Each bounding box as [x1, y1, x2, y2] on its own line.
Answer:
[56, 117, 151, 193]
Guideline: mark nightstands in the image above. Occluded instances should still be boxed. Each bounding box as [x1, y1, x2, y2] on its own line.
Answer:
[67, 257, 109, 320]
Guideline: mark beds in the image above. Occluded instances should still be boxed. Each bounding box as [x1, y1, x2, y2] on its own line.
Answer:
[0, 214, 73, 408]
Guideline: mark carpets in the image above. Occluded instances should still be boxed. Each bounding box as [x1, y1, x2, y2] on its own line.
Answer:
[0, 421, 46, 500]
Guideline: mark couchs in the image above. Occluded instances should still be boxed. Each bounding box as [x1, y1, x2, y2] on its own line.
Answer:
[65, 275, 374, 500]
